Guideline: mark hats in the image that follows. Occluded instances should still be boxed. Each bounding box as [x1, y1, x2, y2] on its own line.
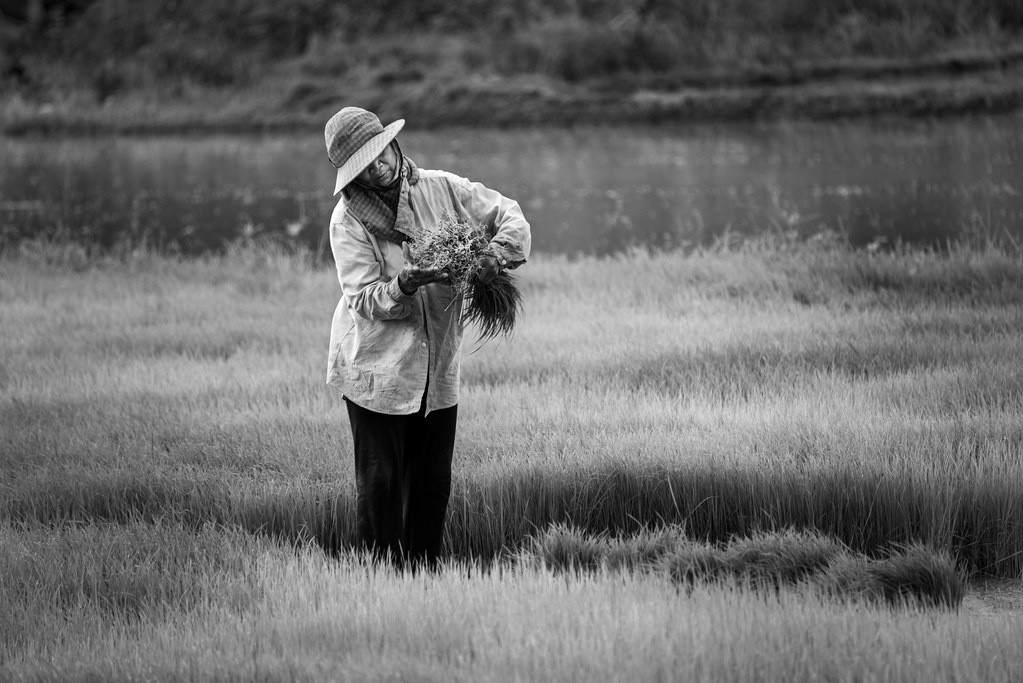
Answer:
[324, 106, 406, 197]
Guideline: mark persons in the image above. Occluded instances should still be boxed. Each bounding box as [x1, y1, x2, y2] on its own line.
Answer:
[325, 107, 531, 574]
